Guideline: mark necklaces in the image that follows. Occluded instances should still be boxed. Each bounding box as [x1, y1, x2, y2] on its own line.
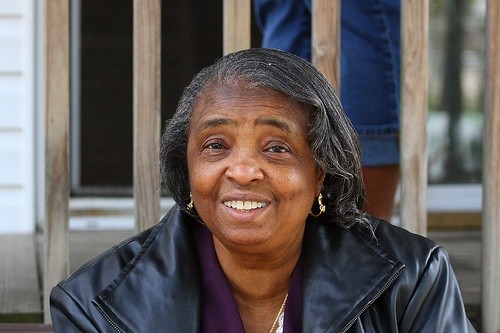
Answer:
[269, 292, 288, 333]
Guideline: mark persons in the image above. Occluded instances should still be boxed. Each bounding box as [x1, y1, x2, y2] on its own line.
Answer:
[254, 0, 402, 227]
[48, 49, 479, 332]
